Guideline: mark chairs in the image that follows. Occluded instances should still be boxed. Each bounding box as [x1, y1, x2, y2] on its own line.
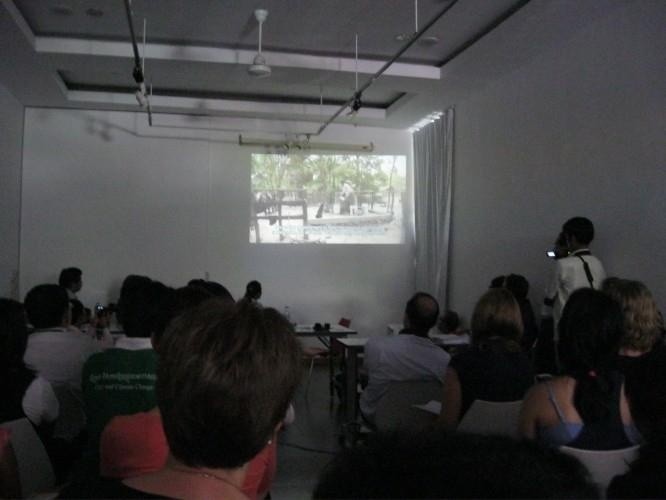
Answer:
[0, 315, 666, 499]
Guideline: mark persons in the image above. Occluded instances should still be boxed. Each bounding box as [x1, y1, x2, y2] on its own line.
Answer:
[545, 217, 606, 342]
[309, 273, 665, 500]
[2, 268, 303, 499]
[340, 179, 357, 214]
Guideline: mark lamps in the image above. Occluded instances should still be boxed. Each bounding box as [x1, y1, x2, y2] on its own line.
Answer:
[131, 32, 143, 87]
[133, 15, 148, 113]
[273, 130, 315, 152]
[348, 32, 363, 117]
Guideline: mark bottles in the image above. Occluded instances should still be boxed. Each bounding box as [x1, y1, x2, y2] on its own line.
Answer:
[282, 305, 289, 320]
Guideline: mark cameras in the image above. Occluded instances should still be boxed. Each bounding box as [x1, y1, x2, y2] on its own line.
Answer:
[546, 234, 569, 257]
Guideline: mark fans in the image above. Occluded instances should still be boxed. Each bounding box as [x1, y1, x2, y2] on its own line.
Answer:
[389, 0, 445, 52]
[244, 6, 273, 78]
